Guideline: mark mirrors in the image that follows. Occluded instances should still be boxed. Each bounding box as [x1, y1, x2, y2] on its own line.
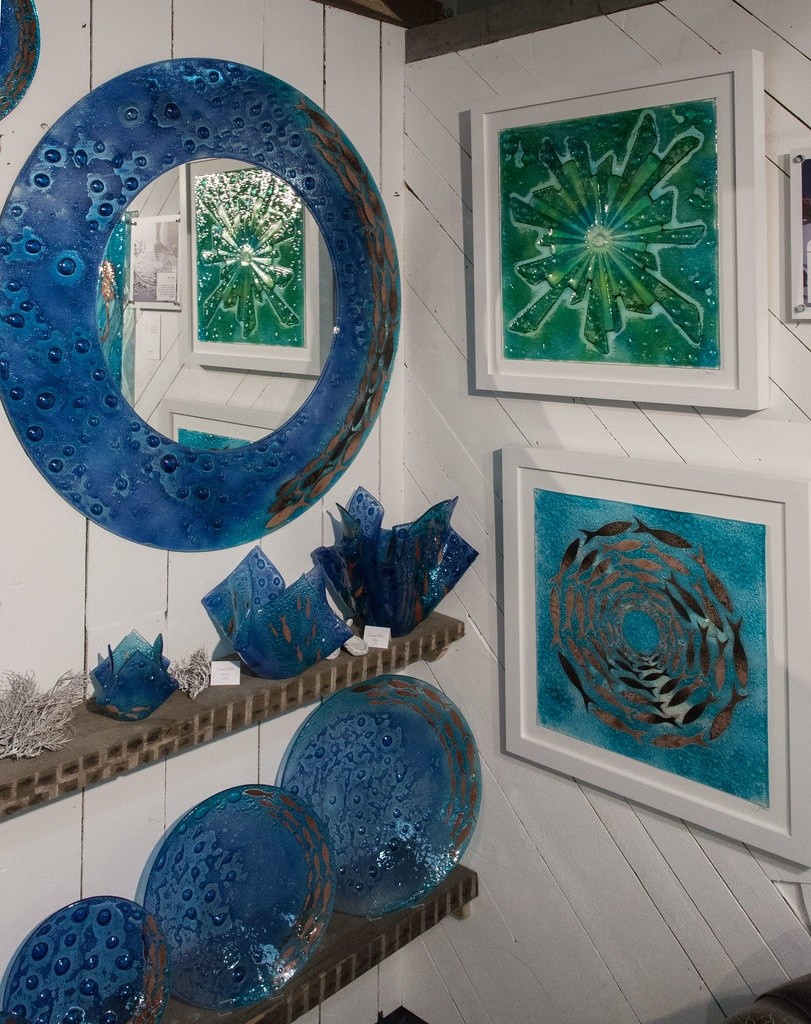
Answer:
[1, 57, 400, 555]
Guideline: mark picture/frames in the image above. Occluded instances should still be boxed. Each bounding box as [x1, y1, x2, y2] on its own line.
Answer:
[501, 444, 811, 869]
[468, 45, 773, 414]
[165, 410, 276, 456]
[789, 146, 811, 322]
[190, 158, 325, 380]
[128, 212, 184, 310]
